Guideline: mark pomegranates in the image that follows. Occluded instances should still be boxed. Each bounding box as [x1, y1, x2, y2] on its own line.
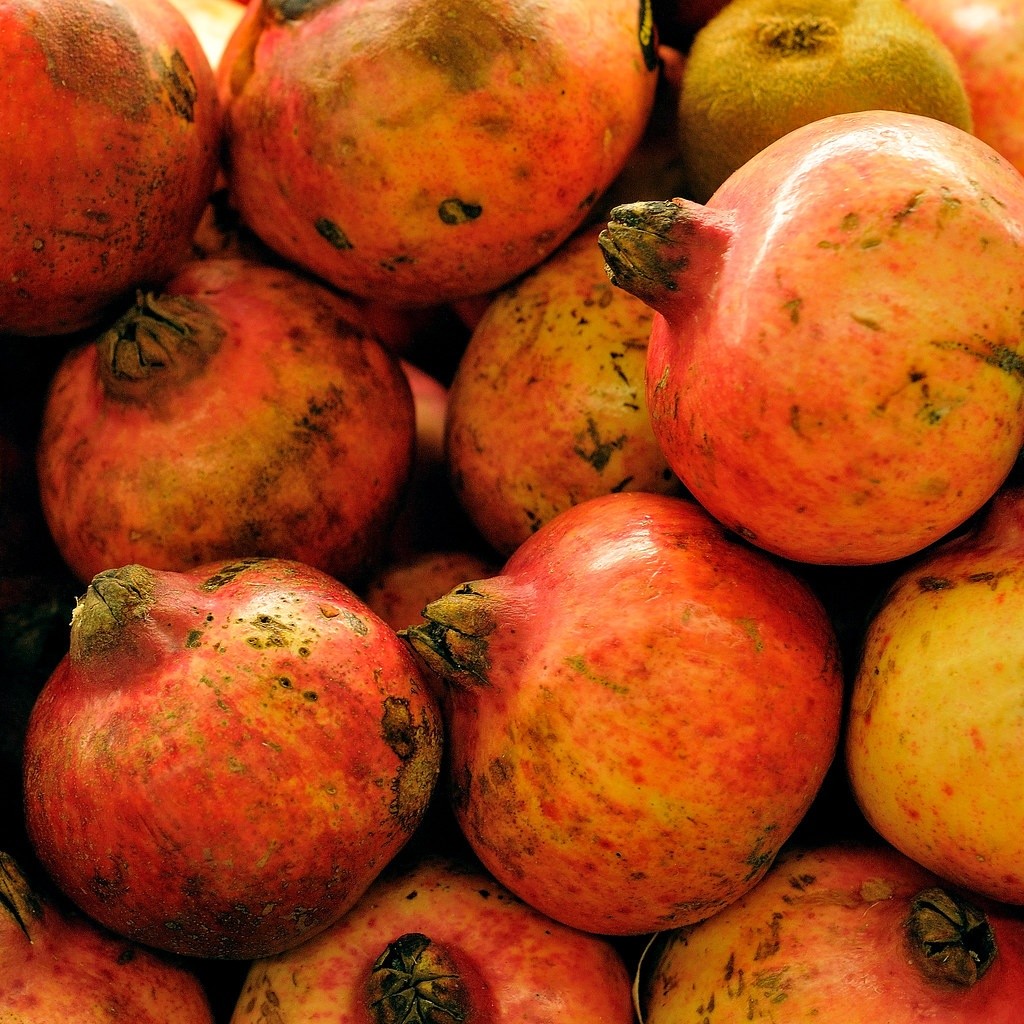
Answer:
[0, 1, 1022, 1024]
